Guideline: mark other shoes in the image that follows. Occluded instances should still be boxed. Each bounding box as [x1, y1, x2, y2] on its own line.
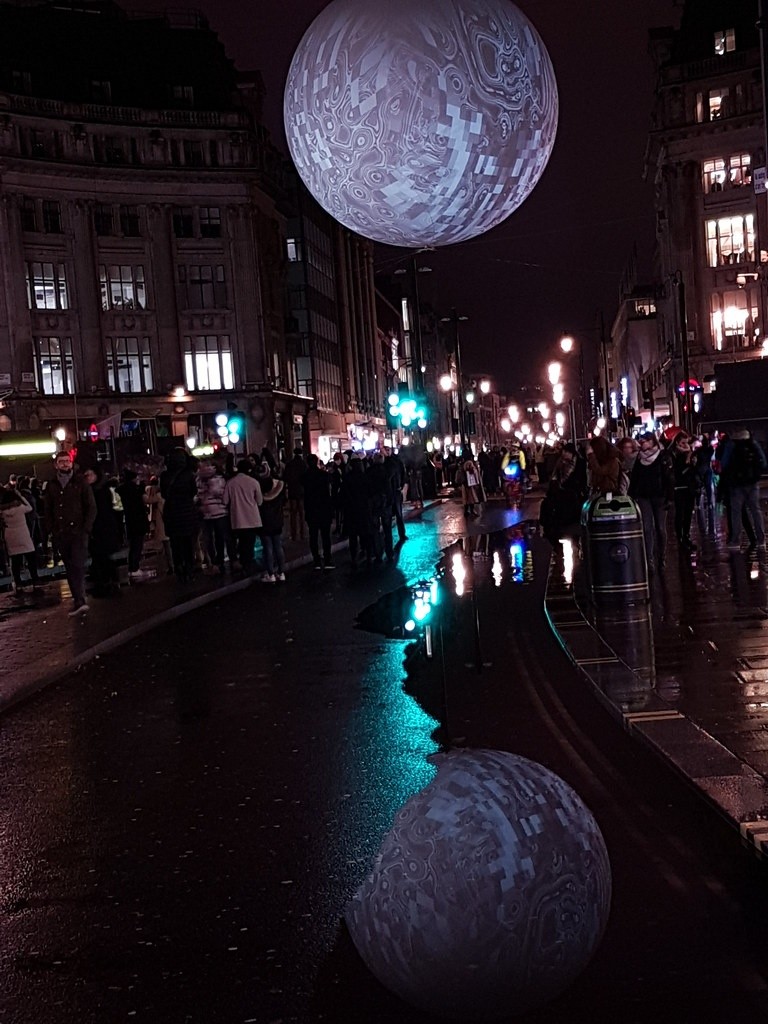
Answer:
[312, 563, 322, 570]
[463, 511, 480, 517]
[323, 562, 337, 570]
[371, 557, 383, 564]
[399, 536, 409, 539]
[167, 559, 258, 579]
[360, 559, 374, 566]
[382, 557, 394, 564]
[14, 586, 46, 596]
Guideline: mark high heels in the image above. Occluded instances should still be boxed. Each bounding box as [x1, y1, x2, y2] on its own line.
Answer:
[67, 602, 90, 619]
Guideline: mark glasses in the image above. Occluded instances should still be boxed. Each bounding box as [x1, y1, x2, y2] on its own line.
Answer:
[384, 448, 390, 451]
[57, 458, 73, 465]
[639, 440, 647, 446]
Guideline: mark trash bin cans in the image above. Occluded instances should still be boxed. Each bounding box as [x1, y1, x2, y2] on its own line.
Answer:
[580, 493, 651, 602]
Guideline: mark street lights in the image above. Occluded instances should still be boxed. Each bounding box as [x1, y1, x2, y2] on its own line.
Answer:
[560, 329, 589, 438]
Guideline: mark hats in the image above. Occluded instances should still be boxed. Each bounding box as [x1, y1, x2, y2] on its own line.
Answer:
[373, 452, 384, 460]
[383, 446, 389, 449]
[639, 430, 656, 441]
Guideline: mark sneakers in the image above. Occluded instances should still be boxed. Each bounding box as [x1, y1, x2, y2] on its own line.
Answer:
[274, 570, 286, 581]
[261, 574, 275, 583]
[126, 569, 150, 579]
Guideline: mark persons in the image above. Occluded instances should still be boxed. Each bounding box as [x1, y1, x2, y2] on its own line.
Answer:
[398, 438, 574, 515]
[541, 425, 768, 567]
[0, 445, 412, 618]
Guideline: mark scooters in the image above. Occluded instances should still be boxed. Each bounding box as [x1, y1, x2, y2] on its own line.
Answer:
[502, 465, 524, 515]
